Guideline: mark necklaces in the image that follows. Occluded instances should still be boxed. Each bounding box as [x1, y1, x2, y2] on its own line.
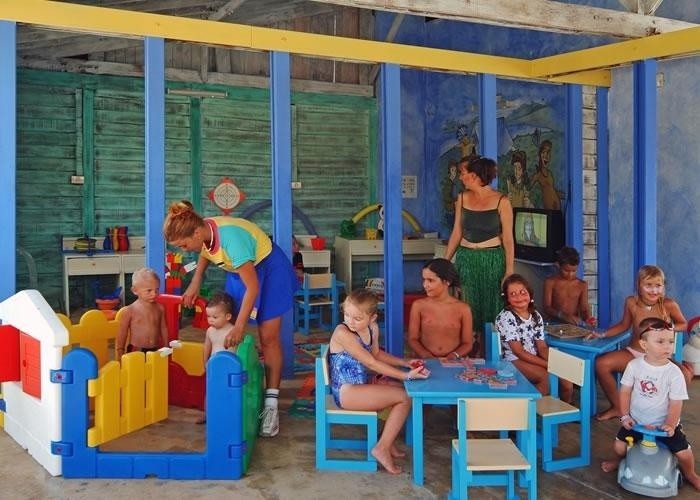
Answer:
[640, 297, 658, 312]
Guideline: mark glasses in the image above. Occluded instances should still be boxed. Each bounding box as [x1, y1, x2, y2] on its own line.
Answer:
[640, 323, 674, 340]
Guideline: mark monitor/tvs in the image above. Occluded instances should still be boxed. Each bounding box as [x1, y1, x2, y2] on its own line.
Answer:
[512, 207, 565, 263]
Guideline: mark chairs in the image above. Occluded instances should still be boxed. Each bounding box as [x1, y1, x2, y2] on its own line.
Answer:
[291, 275, 386, 337]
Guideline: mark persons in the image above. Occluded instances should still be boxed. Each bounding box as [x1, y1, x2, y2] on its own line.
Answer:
[328, 287, 430, 475]
[541, 246, 598, 391]
[497, 273, 581, 424]
[581, 261, 691, 425]
[114, 266, 168, 361]
[291, 235, 304, 288]
[439, 154, 516, 357]
[523, 214, 539, 243]
[162, 198, 298, 439]
[408, 256, 478, 439]
[599, 316, 700, 494]
[191, 290, 236, 428]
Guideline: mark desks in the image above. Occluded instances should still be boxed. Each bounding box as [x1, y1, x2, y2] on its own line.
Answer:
[63, 237, 166, 317]
[291, 232, 457, 297]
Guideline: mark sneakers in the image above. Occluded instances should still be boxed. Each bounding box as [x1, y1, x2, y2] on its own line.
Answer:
[259, 407, 279, 437]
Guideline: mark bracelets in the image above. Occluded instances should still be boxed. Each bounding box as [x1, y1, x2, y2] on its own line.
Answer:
[599, 331, 606, 339]
[451, 351, 459, 358]
[407, 370, 413, 382]
[115, 347, 125, 352]
[619, 415, 631, 421]
